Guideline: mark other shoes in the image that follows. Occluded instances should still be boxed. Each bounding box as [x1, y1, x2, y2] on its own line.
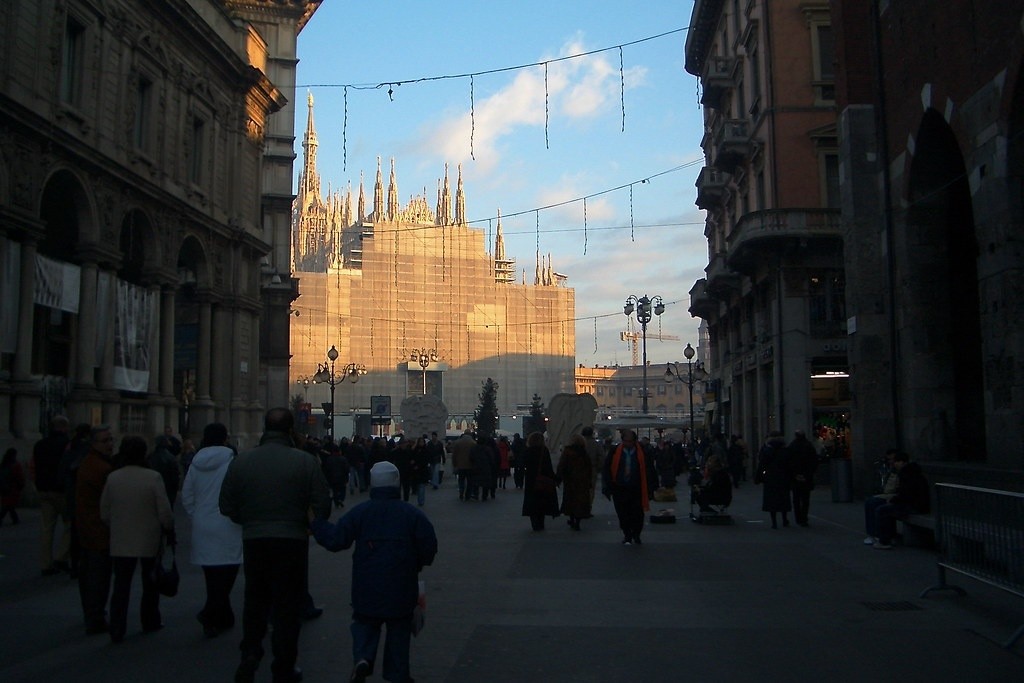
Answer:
[233, 654, 259, 683]
[349, 658, 370, 683]
[42, 566, 61, 575]
[623, 531, 631, 543]
[144, 622, 165, 632]
[872, 541, 892, 550]
[87, 610, 110, 634]
[55, 561, 74, 573]
[305, 606, 322, 620]
[112, 632, 128, 644]
[631, 531, 641, 544]
[393, 677, 415, 683]
[567, 518, 574, 528]
[864, 536, 879, 545]
[891, 538, 897, 545]
[273, 667, 302, 683]
[574, 523, 580, 530]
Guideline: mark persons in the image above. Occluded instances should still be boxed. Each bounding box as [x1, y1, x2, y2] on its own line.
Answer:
[165, 426, 195, 466]
[225, 435, 238, 456]
[0, 448, 25, 524]
[311, 461, 438, 683]
[814, 430, 824, 454]
[602, 430, 651, 545]
[30, 418, 176, 644]
[298, 432, 447, 506]
[181, 423, 242, 635]
[219, 408, 331, 683]
[864, 449, 923, 549]
[755, 428, 818, 528]
[640, 434, 750, 514]
[452, 429, 526, 500]
[522, 427, 602, 531]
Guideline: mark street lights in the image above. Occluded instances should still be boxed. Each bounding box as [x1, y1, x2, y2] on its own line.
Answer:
[623, 293, 667, 413]
[662, 342, 709, 444]
[409, 346, 442, 397]
[313, 342, 360, 441]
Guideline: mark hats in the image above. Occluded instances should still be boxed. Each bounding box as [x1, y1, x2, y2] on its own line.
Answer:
[370, 461, 400, 488]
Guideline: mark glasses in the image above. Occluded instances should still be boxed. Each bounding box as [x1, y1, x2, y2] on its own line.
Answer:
[94, 437, 114, 444]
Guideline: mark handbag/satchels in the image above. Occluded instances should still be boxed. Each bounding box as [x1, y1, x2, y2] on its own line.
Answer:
[411, 580, 426, 636]
[153, 529, 178, 597]
[536, 475, 553, 494]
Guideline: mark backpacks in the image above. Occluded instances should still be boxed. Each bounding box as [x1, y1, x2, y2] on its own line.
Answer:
[0, 463, 12, 494]
